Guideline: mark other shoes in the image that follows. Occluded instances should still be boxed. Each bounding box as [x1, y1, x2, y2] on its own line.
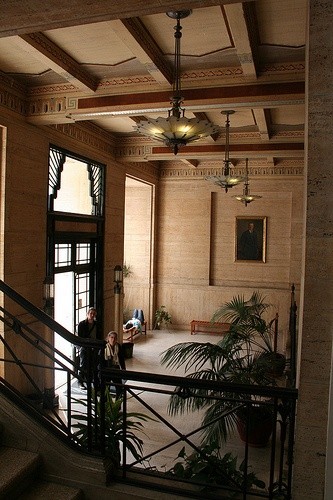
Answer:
[77, 382, 87, 390]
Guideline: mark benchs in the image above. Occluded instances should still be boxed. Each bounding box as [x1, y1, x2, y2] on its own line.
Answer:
[190, 320, 232, 336]
[123, 322, 147, 341]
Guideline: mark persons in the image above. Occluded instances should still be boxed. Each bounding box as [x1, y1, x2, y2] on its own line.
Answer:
[78, 308, 104, 390]
[99, 331, 127, 411]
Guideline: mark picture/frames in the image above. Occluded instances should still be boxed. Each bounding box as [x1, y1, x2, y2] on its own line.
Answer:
[234, 215, 268, 264]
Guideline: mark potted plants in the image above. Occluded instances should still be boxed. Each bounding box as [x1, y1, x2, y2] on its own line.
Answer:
[74, 385, 123, 483]
[165, 342, 275, 447]
[212, 294, 286, 375]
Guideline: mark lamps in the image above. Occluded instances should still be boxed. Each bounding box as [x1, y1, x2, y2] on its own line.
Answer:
[230, 156, 263, 206]
[44, 277, 54, 300]
[132, 9, 226, 155]
[114, 266, 124, 282]
[201, 109, 252, 193]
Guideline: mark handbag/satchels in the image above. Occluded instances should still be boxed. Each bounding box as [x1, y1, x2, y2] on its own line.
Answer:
[79, 346, 85, 358]
